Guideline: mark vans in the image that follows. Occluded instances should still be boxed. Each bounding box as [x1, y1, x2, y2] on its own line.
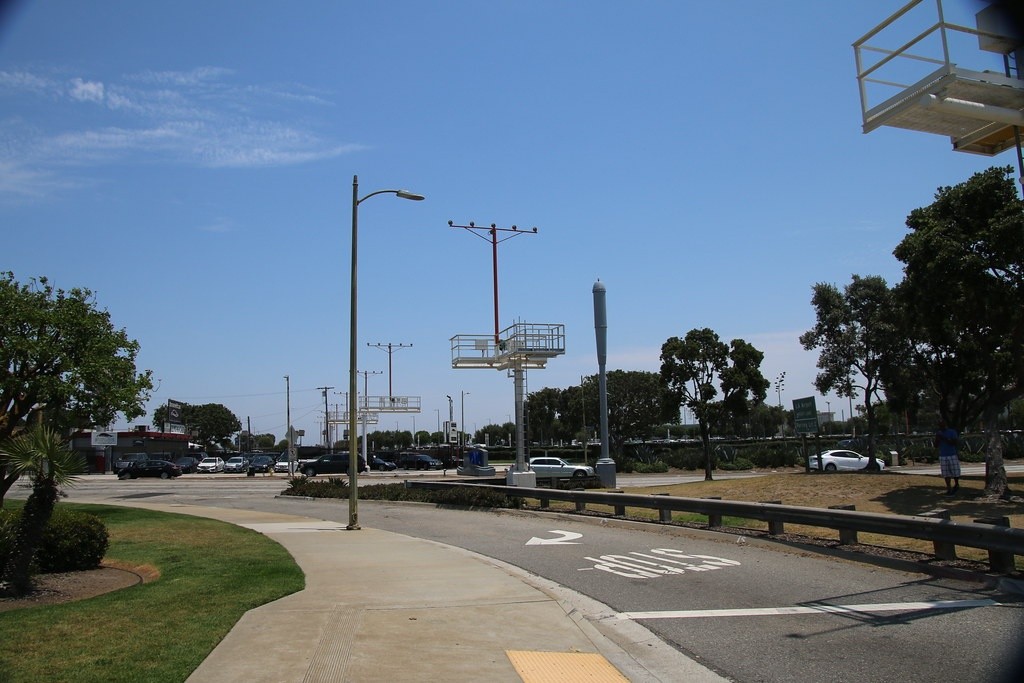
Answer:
[240, 453, 280, 465]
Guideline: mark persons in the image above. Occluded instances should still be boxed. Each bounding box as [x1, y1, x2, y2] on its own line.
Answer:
[935, 419, 961, 494]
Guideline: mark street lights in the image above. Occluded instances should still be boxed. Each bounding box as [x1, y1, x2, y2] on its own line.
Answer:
[461, 390, 471, 450]
[345, 173, 427, 531]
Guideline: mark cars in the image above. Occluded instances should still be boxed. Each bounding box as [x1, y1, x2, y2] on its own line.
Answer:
[118, 461, 183, 479]
[368, 458, 397, 471]
[149, 453, 173, 461]
[839, 440, 858, 449]
[510, 457, 595, 478]
[196, 457, 225, 473]
[248, 456, 274, 472]
[401, 454, 443, 469]
[224, 456, 249, 472]
[808, 450, 885, 474]
[186, 452, 208, 461]
[274, 458, 298, 472]
[175, 456, 199, 473]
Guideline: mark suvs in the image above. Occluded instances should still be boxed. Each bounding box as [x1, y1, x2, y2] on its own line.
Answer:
[299, 453, 366, 477]
[113, 453, 148, 474]
